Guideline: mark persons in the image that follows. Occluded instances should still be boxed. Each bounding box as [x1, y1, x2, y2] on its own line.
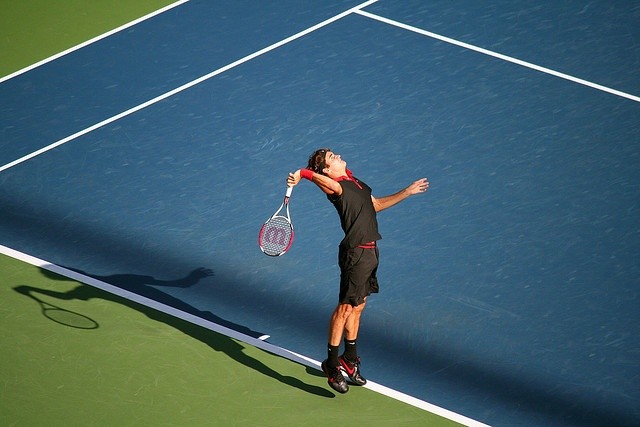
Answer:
[285, 149, 429, 393]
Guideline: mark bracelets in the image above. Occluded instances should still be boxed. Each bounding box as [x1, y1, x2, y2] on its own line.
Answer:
[300, 168, 314, 182]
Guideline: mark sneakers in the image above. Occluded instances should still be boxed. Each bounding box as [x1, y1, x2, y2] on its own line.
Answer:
[339, 353, 366, 386]
[322, 358, 349, 394]
